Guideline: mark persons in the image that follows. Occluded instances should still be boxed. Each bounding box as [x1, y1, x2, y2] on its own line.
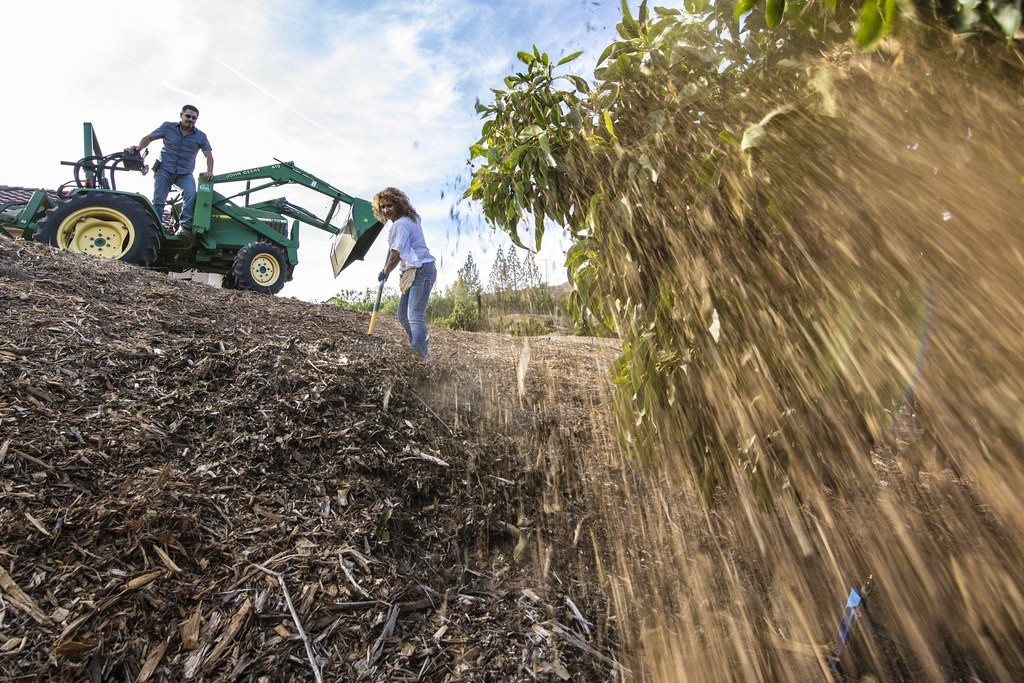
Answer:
[126, 104, 215, 239]
[371, 187, 439, 357]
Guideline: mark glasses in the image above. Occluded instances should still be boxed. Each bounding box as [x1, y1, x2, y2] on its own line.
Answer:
[380, 202, 394, 209]
[182, 113, 197, 120]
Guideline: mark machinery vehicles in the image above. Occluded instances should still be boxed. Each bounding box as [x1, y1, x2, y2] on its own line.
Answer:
[0, 121, 388, 296]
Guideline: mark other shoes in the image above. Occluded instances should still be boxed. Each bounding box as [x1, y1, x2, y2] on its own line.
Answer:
[175, 225, 190, 241]
